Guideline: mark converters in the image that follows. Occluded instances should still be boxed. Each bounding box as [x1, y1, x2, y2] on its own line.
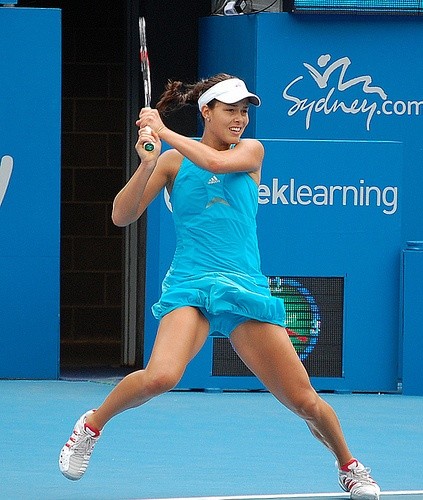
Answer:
[234, 0, 247, 13]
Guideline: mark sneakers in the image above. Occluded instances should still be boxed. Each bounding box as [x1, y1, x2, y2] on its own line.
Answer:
[335, 458, 380, 500]
[59, 409, 104, 481]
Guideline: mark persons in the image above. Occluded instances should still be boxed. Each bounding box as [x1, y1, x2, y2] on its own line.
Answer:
[58, 73, 382, 500]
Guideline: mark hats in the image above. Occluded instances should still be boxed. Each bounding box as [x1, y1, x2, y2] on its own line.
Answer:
[198, 78, 261, 111]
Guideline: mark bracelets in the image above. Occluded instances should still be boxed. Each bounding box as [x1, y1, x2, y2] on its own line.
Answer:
[155, 124, 165, 134]
